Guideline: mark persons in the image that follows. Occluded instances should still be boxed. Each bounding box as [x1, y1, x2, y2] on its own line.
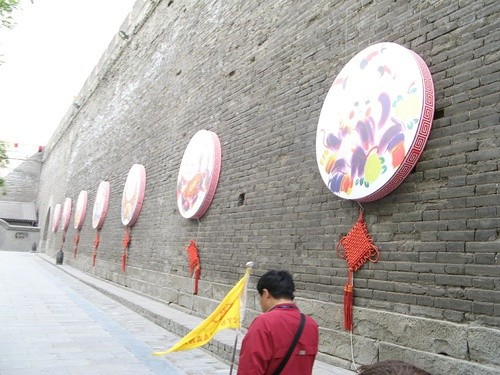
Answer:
[237, 270, 318, 375]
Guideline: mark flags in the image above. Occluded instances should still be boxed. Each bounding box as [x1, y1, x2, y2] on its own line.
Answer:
[152, 267, 252, 355]
